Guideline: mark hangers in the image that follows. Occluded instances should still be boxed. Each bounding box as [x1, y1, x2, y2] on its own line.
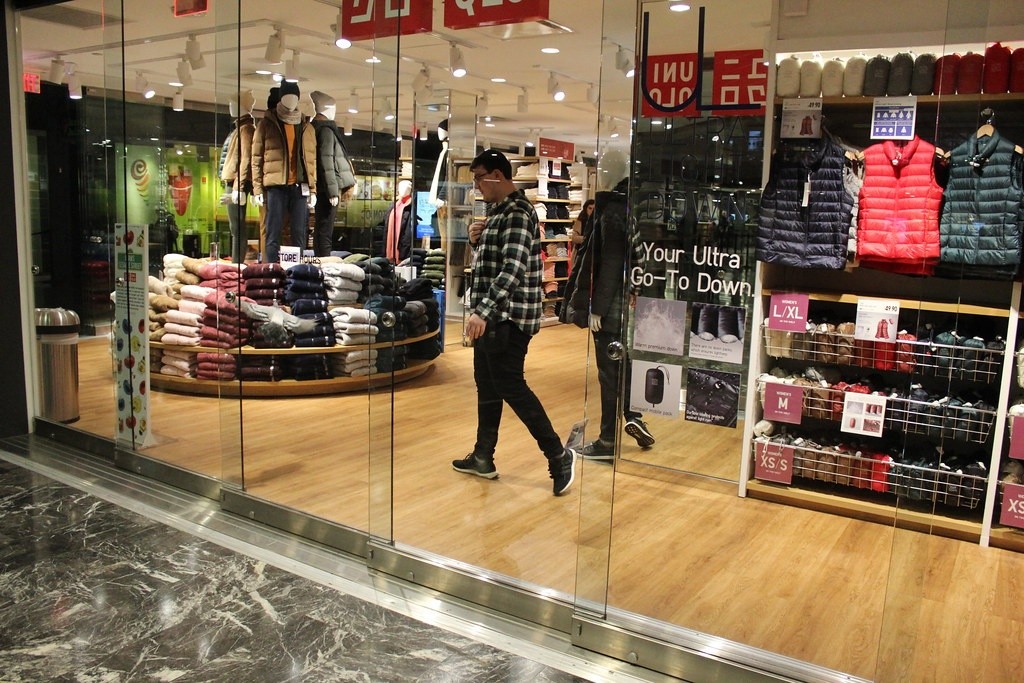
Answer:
[773, 115, 864, 161]
[943, 107, 1023, 158]
[858, 137, 945, 159]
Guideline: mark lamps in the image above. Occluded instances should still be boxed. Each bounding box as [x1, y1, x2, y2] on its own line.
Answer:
[475, 90, 488, 115]
[395, 128, 403, 141]
[549, 72, 566, 102]
[517, 87, 529, 113]
[382, 99, 394, 119]
[449, 40, 466, 77]
[616, 45, 635, 78]
[177, 56, 192, 85]
[49, 53, 66, 85]
[137, 73, 155, 100]
[331, 6, 352, 49]
[410, 62, 434, 105]
[67, 69, 82, 99]
[343, 117, 352, 136]
[608, 116, 618, 138]
[283, 49, 300, 83]
[186, 33, 205, 70]
[587, 83, 602, 109]
[347, 89, 360, 114]
[173, 86, 184, 111]
[266, 25, 284, 64]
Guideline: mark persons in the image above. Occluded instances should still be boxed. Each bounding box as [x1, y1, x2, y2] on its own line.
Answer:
[381, 180, 412, 264]
[219, 90, 254, 263]
[623, 361, 655, 447]
[451, 148, 577, 493]
[573, 199, 595, 246]
[430, 118, 448, 249]
[252, 80, 308, 261]
[557, 149, 629, 460]
[308, 90, 356, 257]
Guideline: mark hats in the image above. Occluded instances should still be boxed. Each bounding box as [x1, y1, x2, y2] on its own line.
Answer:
[279, 78, 300, 99]
[267, 86, 282, 109]
[310, 91, 335, 113]
[232, 91, 256, 113]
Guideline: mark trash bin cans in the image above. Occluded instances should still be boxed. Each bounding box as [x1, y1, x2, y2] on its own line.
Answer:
[36, 308, 82, 424]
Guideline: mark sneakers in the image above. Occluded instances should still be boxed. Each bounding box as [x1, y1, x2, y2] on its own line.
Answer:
[548, 448, 576, 495]
[577, 440, 615, 459]
[624, 418, 655, 447]
[452, 453, 497, 478]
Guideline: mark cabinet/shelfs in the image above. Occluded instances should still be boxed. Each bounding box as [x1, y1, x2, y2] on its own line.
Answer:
[448, 155, 587, 327]
[739, 1, 1023, 552]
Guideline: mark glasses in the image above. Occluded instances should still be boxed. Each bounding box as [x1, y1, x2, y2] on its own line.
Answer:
[473, 173, 487, 183]
